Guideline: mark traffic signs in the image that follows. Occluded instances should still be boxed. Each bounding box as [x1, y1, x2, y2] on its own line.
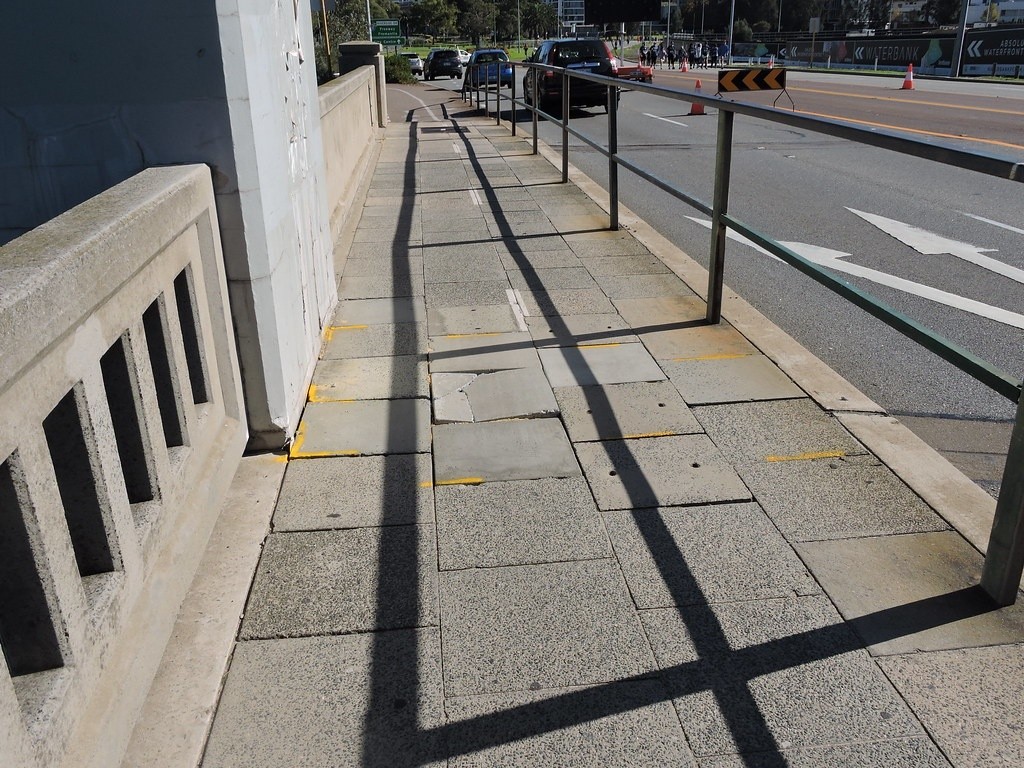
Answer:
[372, 38, 405, 46]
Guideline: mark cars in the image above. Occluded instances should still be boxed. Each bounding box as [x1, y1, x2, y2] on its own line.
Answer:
[465, 49, 513, 91]
[422, 48, 464, 80]
[400, 53, 423, 76]
[459, 49, 472, 67]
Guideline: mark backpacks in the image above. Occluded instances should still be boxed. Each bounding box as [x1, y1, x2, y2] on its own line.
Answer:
[702, 48, 708, 56]
[690, 49, 695, 55]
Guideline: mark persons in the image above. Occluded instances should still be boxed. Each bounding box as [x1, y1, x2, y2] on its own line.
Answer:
[639, 40, 729, 69]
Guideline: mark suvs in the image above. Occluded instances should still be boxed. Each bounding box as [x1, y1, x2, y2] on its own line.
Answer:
[521, 37, 621, 120]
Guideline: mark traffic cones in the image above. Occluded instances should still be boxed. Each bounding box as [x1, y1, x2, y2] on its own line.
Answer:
[686, 80, 707, 116]
[638, 61, 641, 67]
[899, 64, 915, 90]
[681, 58, 688, 73]
[768, 61, 773, 68]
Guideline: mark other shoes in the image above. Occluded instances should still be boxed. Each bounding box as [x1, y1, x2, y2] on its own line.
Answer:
[667, 66, 725, 69]
